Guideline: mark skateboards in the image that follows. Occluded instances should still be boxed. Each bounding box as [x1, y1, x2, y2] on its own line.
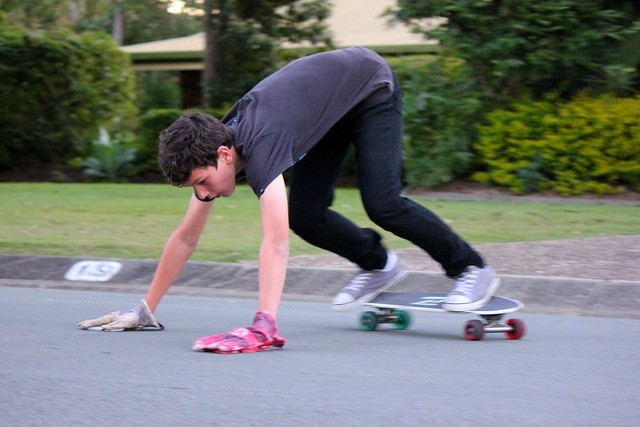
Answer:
[359, 291, 527, 340]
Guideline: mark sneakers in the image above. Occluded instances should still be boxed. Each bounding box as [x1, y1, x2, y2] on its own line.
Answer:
[331, 249, 408, 312]
[440, 264, 502, 311]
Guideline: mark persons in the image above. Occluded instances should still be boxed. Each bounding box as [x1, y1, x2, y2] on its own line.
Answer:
[78, 45, 503, 354]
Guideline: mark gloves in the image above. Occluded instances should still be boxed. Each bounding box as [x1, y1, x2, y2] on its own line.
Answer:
[78, 299, 160, 330]
[192, 311, 279, 351]
